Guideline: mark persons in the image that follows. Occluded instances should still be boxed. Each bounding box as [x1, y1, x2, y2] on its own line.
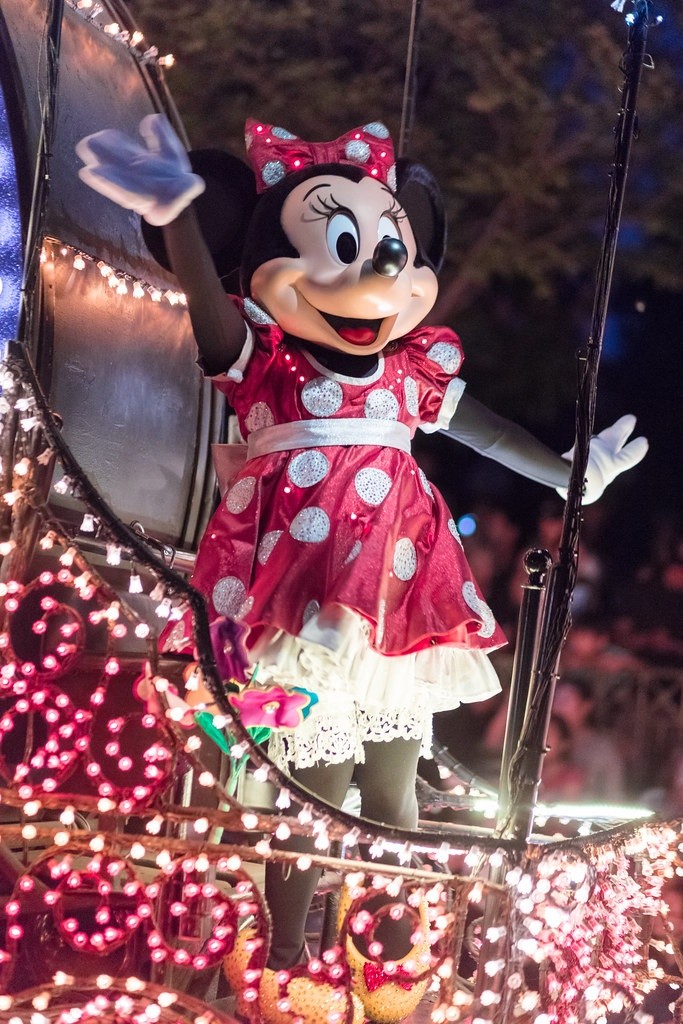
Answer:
[428, 490, 681, 1023]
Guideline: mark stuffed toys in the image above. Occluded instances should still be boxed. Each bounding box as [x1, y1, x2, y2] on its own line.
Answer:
[72, 110, 651, 1024]
